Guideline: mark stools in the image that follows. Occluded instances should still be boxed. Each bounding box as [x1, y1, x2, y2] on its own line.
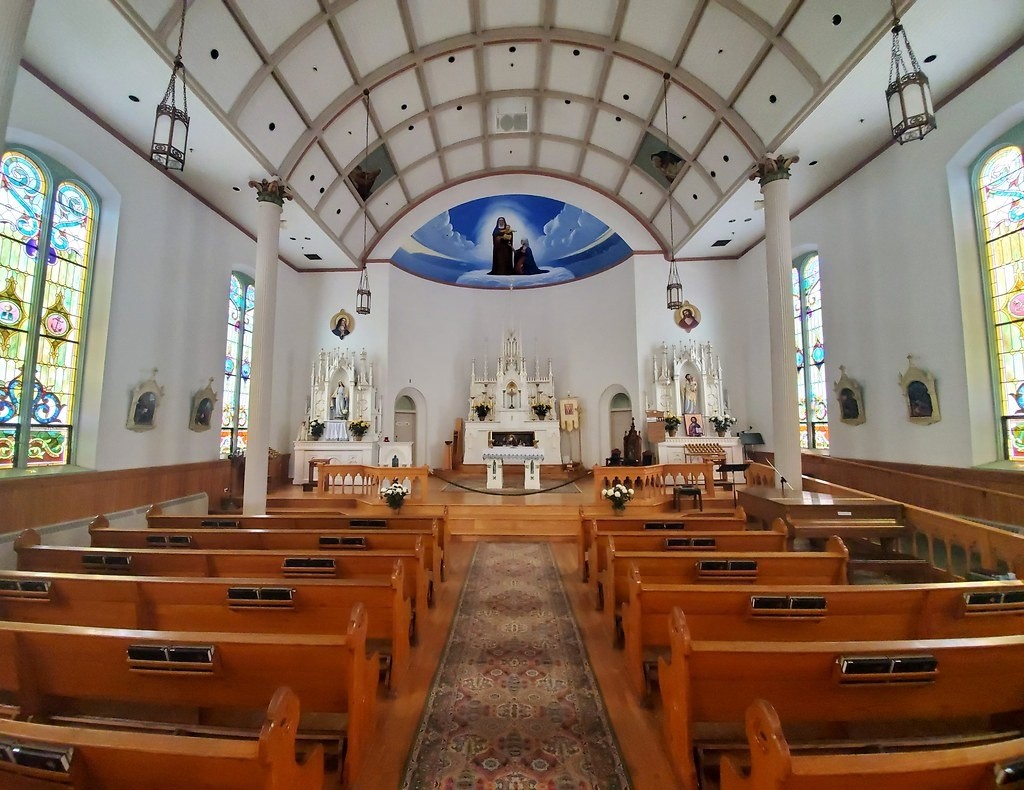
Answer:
[673, 488, 702, 513]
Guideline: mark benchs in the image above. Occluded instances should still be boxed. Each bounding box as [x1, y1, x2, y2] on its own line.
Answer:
[579, 506, 1024, 789]
[1, 505, 448, 790]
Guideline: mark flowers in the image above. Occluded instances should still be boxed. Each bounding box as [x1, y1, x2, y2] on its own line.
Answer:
[472, 402, 493, 417]
[380, 483, 409, 510]
[664, 413, 681, 431]
[348, 420, 370, 437]
[531, 402, 553, 415]
[709, 414, 736, 432]
[602, 483, 634, 506]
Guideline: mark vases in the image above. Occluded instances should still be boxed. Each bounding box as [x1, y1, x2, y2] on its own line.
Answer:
[612, 503, 625, 516]
[539, 414, 544, 420]
[480, 415, 485, 421]
[669, 430, 676, 437]
[390, 506, 400, 515]
[355, 433, 362, 441]
[719, 431, 725, 437]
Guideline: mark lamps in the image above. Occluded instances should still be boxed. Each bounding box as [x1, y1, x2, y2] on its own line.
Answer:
[886, 0, 937, 145]
[149, 0, 190, 172]
[356, 88, 371, 315]
[663, 72, 683, 309]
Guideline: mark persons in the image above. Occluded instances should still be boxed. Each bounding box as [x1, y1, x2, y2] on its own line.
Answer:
[683, 374, 697, 413]
[331, 380, 349, 418]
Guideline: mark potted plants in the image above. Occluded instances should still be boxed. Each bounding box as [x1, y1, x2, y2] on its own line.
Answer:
[310, 420, 325, 440]
